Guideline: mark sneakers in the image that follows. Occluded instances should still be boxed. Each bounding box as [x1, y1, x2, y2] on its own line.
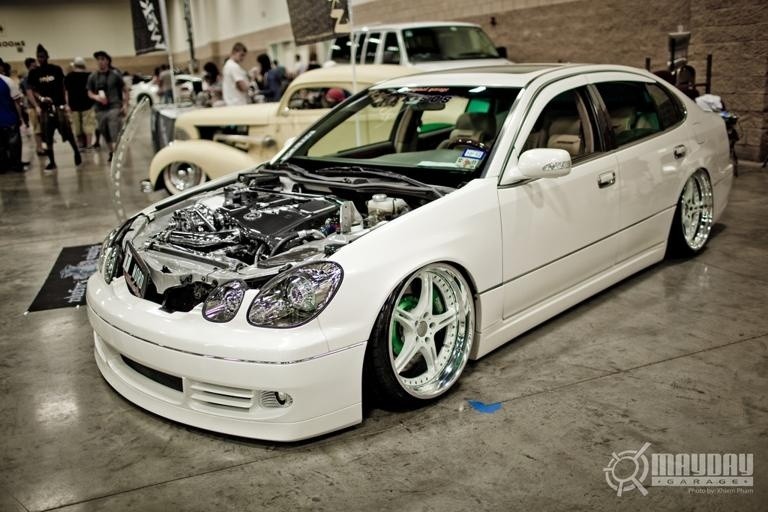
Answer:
[1, 139, 113, 173]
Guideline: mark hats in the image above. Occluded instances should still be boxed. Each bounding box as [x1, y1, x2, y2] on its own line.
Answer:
[74, 57, 85, 66]
[94, 51, 111, 64]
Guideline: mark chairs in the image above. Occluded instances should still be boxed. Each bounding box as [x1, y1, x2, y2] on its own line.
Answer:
[437, 107, 657, 164]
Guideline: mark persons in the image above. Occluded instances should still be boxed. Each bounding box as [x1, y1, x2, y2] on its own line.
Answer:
[0, 42, 348, 174]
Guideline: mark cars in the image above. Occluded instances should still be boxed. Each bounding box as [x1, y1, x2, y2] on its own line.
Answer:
[86, 21, 733, 445]
